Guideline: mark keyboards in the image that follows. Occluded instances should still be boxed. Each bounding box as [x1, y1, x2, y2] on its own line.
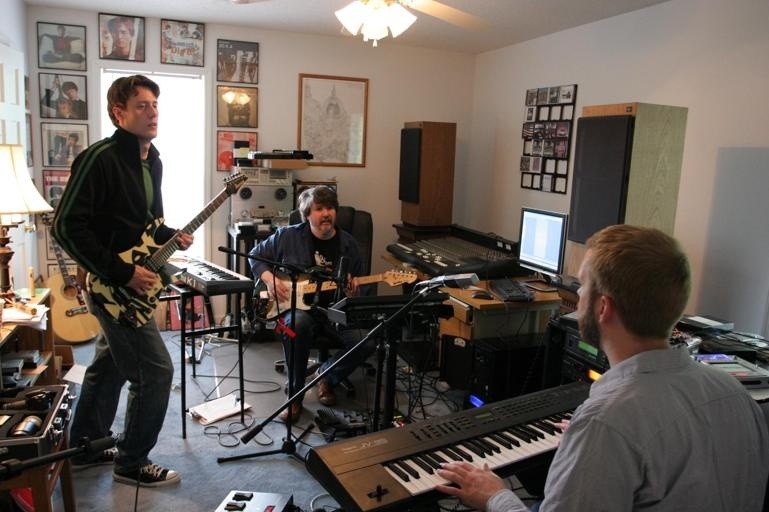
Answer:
[490, 279, 535, 303]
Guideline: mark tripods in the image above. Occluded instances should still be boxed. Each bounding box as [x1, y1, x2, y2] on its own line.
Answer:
[217, 244, 337, 473]
[186, 294, 239, 364]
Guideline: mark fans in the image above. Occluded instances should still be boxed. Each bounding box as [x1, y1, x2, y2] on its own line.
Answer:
[236, 0, 486, 37]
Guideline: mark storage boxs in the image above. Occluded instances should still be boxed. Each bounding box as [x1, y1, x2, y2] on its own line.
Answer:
[51, 343, 89, 406]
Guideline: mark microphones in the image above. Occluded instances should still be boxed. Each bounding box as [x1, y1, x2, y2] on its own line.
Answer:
[417, 273, 479, 287]
[338, 255, 348, 290]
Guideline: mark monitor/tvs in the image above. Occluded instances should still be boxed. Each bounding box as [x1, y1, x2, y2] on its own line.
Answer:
[516, 207, 568, 292]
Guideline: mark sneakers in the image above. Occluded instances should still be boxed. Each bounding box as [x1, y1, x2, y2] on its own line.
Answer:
[279, 393, 305, 423]
[68, 447, 120, 472]
[112, 461, 182, 490]
[314, 366, 339, 408]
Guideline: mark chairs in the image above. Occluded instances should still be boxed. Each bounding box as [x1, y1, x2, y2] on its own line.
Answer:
[273, 204, 377, 398]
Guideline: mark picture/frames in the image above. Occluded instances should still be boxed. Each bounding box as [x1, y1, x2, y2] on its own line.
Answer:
[45, 224, 75, 260]
[46, 263, 79, 278]
[517, 83, 580, 194]
[296, 72, 370, 169]
[159, 18, 206, 68]
[37, 72, 89, 122]
[216, 38, 259, 85]
[97, 12, 146, 62]
[39, 121, 89, 168]
[216, 130, 258, 172]
[0, 62, 35, 222]
[216, 85, 259, 129]
[40, 169, 73, 223]
[36, 21, 88, 72]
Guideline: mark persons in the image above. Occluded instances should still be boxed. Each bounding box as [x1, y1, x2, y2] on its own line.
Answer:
[60, 132, 83, 166]
[55, 98, 79, 119]
[39, 26, 84, 64]
[40, 76, 87, 119]
[49, 75, 193, 487]
[248, 183, 375, 423]
[102, 16, 137, 59]
[429, 225, 768, 511]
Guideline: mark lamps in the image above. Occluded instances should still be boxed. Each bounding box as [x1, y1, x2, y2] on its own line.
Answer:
[333, 0, 419, 45]
[0, 141, 55, 297]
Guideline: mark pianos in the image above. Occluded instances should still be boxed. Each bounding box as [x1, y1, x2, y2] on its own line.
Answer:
[162, 254, 253, 297]
[306, 379, 594, 511]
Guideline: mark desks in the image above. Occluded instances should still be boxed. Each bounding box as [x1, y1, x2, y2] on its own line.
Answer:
[224, 225, 286, 344]
[381, 250, 563, 392]
[163, 279, 247, 441]
[0, 427, 79, 511]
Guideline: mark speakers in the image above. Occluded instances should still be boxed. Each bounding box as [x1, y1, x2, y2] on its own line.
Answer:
[565, 102, 689, 285]
[399, 120, 458, 225]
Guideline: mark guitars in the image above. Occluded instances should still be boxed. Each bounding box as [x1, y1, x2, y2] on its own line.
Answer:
[85, 172, 247, 330]
[251, 266, 418, 323]
[55, 74, 79, 118]
[41, 214, 101, 343]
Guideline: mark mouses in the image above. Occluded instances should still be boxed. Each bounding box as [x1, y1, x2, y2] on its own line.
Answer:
[471, 291, 493, 300]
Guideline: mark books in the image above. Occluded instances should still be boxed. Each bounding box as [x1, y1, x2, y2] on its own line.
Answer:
[0, 303, 50, 389]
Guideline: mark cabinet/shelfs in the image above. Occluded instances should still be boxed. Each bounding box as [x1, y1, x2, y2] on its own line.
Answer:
[0, 287, 58, 511]
